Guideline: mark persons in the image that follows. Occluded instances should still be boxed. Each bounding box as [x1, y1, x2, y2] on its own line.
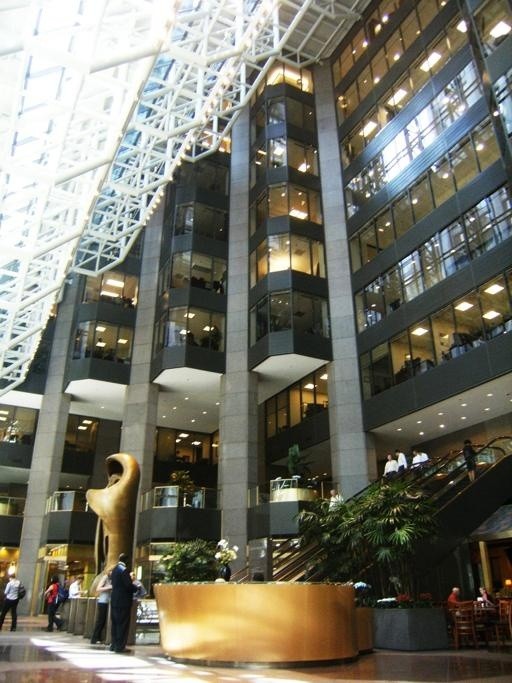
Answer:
[328, 489, 344, 512]
[448, 587, 473, 610]
[463, 440, 476, 481]
[383, 449, 429, 476]
[480, 587, 498, 607]
[0, 552, 147, 653]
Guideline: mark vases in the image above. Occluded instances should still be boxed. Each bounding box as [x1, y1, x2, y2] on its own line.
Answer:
[218, 564, 231, 580]
[400, 602, 409, 608]
[421, 601, 432, 607]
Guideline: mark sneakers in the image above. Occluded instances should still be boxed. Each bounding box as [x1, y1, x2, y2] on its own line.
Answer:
[91, 639, 134, 654]
[43, 619, 65, 632]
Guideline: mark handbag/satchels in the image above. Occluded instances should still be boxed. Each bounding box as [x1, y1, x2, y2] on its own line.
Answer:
[18, 584, 27, 599]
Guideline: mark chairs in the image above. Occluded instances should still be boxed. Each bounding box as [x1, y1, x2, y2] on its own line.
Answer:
[450, 600, 512, 650]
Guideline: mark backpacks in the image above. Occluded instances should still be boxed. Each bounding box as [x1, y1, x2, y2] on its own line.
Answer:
[56, 586, 70, 602]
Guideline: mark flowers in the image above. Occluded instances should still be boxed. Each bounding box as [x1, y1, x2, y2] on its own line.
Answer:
[215, 539, 238, 565]
[420, 593, 433, 600]
[397, 595, 409, 601]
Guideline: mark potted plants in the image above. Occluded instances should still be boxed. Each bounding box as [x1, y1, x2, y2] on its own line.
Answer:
[342, 475, 449, 652]
